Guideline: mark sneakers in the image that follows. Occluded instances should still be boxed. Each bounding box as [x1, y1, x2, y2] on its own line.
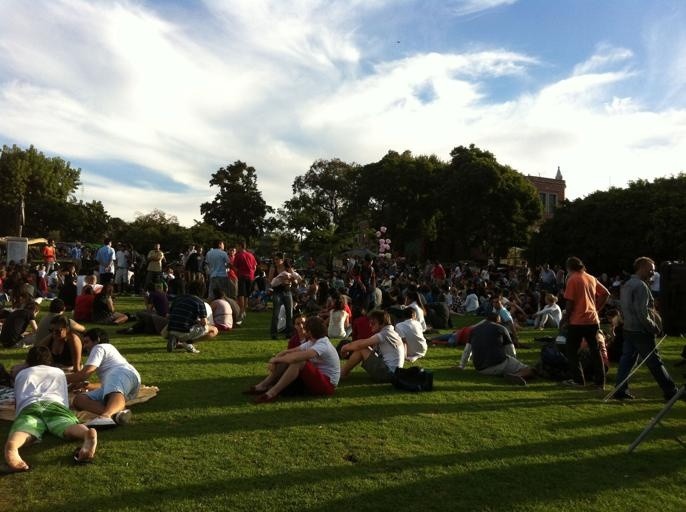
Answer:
[115, 407, 133, 427]
[166, 335, 177, 353]
[84, 415, 117, 427]
[583, 383, 605, 392]
[663, 389, 686, 401]
[182, 342, 201, 354]
[613, 390, 636, 399]
[509, 373, 527, 386]
[561, 378, 585, 389]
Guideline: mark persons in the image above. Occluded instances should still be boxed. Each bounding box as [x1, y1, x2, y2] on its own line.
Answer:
[0, 236, 686, 473]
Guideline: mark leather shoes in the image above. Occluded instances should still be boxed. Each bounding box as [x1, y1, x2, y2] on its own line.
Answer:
[250, 391, 278, 405]
[240, 382, 269, 398]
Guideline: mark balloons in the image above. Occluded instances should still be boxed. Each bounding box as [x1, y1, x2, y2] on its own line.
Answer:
[376, 231, 381, 237]
[380, 227, 387, 232]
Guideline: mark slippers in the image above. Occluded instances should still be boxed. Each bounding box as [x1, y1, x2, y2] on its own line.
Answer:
[0, 460, 32, 477]
[72, 446, 95, 464]
